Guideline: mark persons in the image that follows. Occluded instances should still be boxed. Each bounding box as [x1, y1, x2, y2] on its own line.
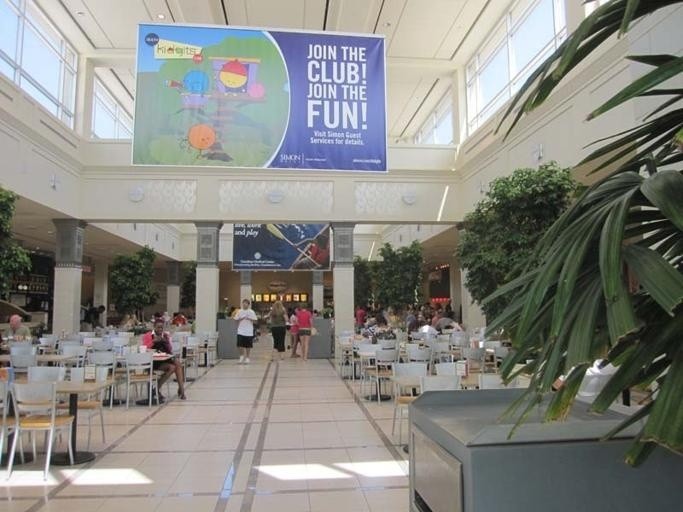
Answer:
[120, 312, 188, 329]
[230, 306, 240, 317]
[269, 300, 319, 361]
[143, 320, 187, 400]
[233, 299, 257, 364]
[2, 314, 31, 338]
[84, 305, 105, 329]
[288, 234, 330, 269]
[355, 299, 464, 370]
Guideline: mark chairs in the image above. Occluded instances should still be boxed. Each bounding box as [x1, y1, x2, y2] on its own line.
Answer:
[0, 327, 221, 480]
[338, 330, 563, 448]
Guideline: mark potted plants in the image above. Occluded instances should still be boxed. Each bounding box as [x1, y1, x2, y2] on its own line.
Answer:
[320, 307, 333, 319]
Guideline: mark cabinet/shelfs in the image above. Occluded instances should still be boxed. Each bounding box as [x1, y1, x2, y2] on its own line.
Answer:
[408, 389, 683, 512]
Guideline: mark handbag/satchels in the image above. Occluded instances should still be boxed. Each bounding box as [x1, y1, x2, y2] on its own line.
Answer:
[150, 341, 167, 352]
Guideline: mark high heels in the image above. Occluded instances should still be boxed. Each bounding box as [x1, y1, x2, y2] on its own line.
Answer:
[177, 388, 186, 400]
[153, 387, 164, 399]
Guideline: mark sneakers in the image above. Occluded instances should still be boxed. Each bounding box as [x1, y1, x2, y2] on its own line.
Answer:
[238, 355, 243, 364]
[244, 357, 250, 364]
